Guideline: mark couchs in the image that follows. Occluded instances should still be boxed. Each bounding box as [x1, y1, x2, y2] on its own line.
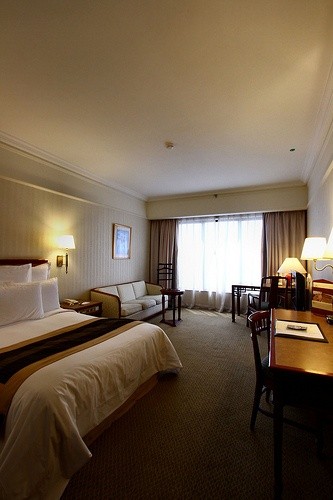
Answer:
[90, 279, 168, 323]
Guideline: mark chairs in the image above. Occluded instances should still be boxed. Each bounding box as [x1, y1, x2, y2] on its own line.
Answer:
[246, 276, 287, 326]
[247, 310, 325, 459]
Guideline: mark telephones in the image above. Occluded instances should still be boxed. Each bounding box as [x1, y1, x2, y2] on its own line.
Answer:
[63, 297, 79, 306]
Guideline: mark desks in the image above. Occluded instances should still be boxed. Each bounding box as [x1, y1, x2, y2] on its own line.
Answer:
[160, 289, 184, 326]
[232, 283, 309, 322]
[269, 308, 333, 500]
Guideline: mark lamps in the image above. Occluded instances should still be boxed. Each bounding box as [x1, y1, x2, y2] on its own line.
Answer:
[278, 258, 307, 287]
[57, 234, 76, 274]
[301, 226, 333, 325]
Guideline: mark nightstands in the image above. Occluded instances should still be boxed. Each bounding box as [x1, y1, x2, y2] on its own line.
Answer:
[60, 299, 103, 317]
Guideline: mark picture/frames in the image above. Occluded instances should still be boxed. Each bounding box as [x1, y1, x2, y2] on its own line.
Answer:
[112, 223, 132, 259]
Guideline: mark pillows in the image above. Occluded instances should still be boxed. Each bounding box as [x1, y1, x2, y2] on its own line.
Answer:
[0, 263, 33, 285]
[32, 262, 50, 282]
[0, 280, 44, 326]
[40, 277, 61, 313]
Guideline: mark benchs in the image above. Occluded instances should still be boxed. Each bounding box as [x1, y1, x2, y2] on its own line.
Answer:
[311, 279, 333, 314]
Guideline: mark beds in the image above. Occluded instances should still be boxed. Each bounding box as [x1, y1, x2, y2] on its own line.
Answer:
[0, 257, 183, 500]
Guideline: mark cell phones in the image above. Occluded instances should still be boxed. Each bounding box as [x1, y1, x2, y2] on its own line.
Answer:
[287, 324, 307, 330]
